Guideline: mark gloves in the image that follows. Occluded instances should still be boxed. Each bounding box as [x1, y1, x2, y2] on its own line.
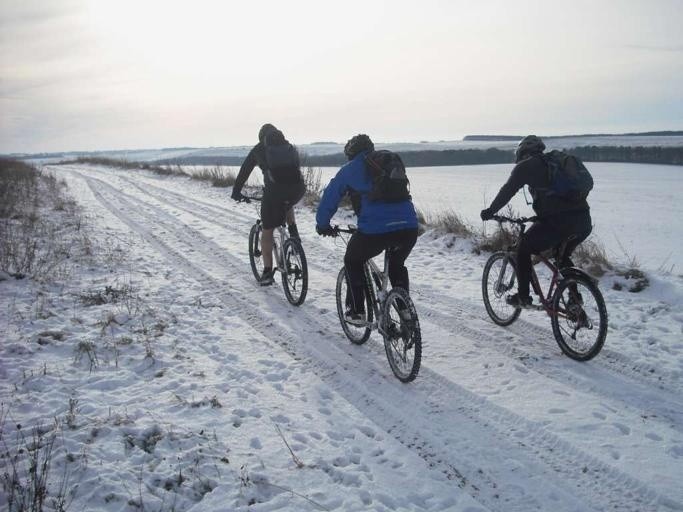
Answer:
[232, 192, 243, 202]
[480, 209, 494, 221]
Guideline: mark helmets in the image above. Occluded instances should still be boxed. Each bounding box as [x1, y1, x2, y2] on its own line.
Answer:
[515, 135, 546, 163]
[344, 134, 374, 155]
[258, 123, 278, 141]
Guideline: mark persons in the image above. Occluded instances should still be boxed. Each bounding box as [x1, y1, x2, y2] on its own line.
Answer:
[317, 134, 418, 324]
[231, 123, 306, 286]
[481, 135, 592, 307]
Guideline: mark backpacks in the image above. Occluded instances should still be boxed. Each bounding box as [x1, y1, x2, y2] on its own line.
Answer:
[267, 131, 299, 174]
[530, 149, 594, 203]
[367, 149, 409, 200]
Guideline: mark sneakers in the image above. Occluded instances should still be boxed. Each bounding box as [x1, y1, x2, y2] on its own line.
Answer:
[506, 294, 532, 309]
[258, 271, 273, 284]
[343, 309, 367, 325]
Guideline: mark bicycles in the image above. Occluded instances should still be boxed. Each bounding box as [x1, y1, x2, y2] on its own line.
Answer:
[482, 213, 609, 362]
[236, 195, 307, 306]
[315, 225, 422, 384]
